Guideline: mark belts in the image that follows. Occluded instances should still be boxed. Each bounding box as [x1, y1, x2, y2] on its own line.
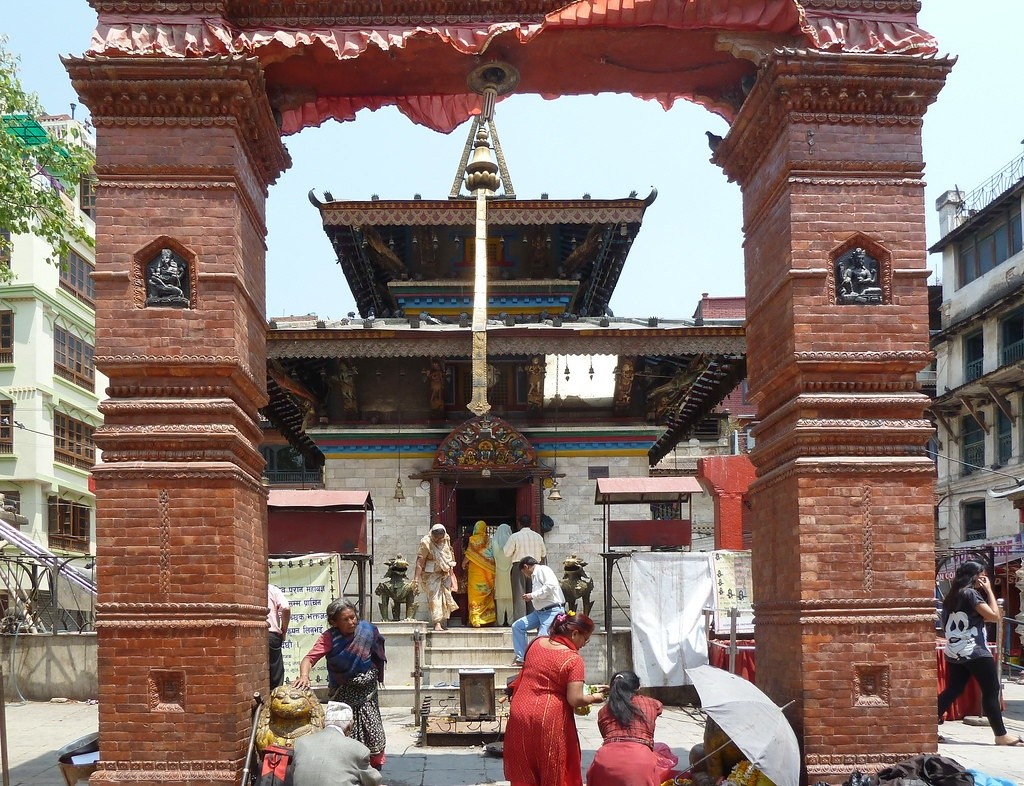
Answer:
[538, 603, 564, 611]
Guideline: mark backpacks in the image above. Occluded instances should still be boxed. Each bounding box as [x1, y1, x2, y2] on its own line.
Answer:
[254, 744, 294, 786]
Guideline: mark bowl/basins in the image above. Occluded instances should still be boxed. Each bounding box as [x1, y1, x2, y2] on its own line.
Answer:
[55, 732, 99, 761]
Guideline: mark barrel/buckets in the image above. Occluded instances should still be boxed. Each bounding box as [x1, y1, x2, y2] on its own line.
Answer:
[58, 758, 97, 786]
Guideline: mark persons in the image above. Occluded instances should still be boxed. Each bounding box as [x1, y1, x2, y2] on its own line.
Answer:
[336, 360, 359, 407]
[453, 515, 547, 628]
[587, 670, 664, 785]
[615, 358, 634, 404]
[835, 245, 886, 304]
[504, 611, 610, 786]
[512, 556, 567, 666]
[527, 355, 542, 395]
[147, 247, 188, 304]
[292, 701, 383, 786]
[266, 583, 291, 688]
[673, 664, 801, 786]
[936, 561, 1024, 746]
[423, 357, 445, 407]
[412, 524, 456, 631]
[293, 597, 388, 772]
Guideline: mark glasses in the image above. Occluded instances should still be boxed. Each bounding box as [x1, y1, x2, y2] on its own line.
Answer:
[572, 628, 590, 643]
[433, 536, 444, 541]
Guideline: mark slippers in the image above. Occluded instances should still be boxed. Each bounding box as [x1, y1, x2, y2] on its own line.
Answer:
[938, 735, 946, 743]
[994, 736, 1024, 747]
